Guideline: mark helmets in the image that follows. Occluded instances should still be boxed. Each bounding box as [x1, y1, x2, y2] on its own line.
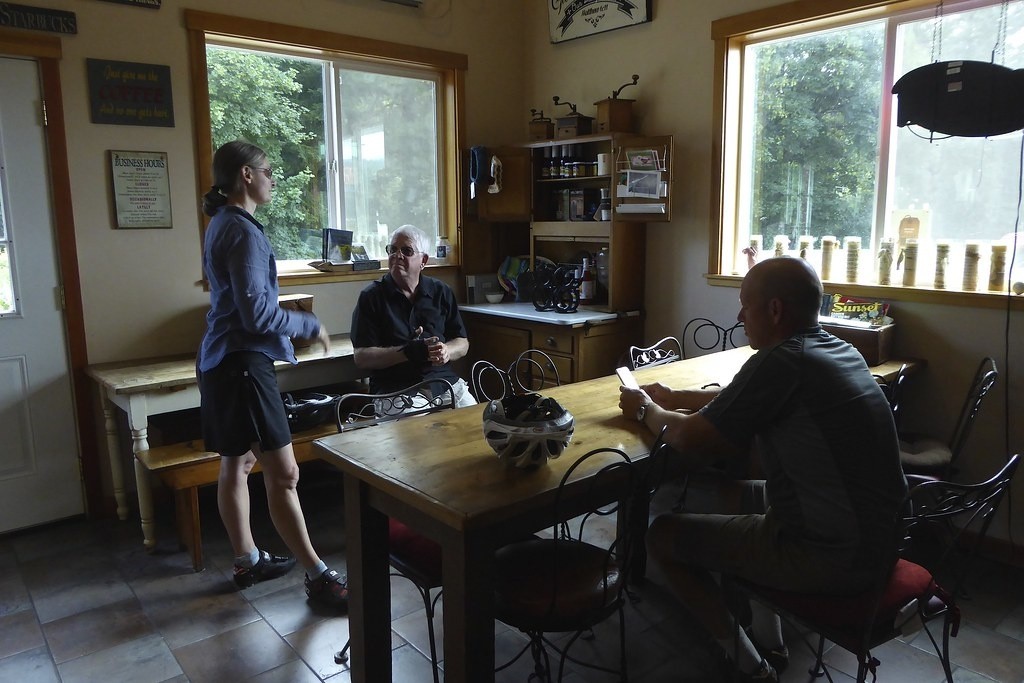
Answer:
[483, 393, 575, 469]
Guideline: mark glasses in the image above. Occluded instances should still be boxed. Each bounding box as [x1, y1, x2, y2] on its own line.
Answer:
[243, 166, 272, 179]
[386, 245, 423, 256]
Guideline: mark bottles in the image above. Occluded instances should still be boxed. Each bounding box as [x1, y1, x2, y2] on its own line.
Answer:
[601, 198, 611, 221]
[434, 236, 452, 266]
[541, 144, 598, 180]
[574, 248, 609, 306]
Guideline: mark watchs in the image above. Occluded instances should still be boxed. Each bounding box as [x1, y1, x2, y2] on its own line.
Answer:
[637, 400, 655, 428]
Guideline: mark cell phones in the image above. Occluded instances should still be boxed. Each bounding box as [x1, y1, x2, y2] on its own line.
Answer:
[615, 366, 640, 390]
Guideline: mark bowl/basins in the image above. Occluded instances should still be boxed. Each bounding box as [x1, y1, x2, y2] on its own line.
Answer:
[485, 293, 504, 304]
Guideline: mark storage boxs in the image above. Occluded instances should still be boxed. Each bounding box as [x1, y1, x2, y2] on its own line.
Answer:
[818, 321, 896, 367]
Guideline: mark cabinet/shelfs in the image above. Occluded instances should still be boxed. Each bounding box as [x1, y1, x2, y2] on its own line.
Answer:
[455, 135, 673, 392]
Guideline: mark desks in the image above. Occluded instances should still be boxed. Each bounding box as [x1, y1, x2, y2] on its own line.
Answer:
[84, 331, 356, 554]
[310, 344, 759, 683]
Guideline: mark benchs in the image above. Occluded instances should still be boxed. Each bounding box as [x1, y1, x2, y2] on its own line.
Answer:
[134, 418, 373, 573]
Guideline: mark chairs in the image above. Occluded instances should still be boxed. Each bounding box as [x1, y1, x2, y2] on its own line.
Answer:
[629, 337, 682, 371]
[491, 420, 667, 683]
[680, 318, 751, 360]
[334, 378, 543, 683]
[471, 349, 560, 405]
[741, 356, 1020, 683]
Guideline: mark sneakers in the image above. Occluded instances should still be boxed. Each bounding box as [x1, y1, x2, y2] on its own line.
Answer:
[232, 549, 297, 589]
[745, 624, 789, 675]
[721, 649, 779, 683]
[304, 567, 349, 615]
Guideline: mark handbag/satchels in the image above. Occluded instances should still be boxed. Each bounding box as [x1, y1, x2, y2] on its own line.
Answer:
[282, 390, 339, 433]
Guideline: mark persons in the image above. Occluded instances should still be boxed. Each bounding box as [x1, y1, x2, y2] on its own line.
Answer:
[619, 255, 914, 683]
[350, 225, 477, 424]
[195, 140, 348, 609]
